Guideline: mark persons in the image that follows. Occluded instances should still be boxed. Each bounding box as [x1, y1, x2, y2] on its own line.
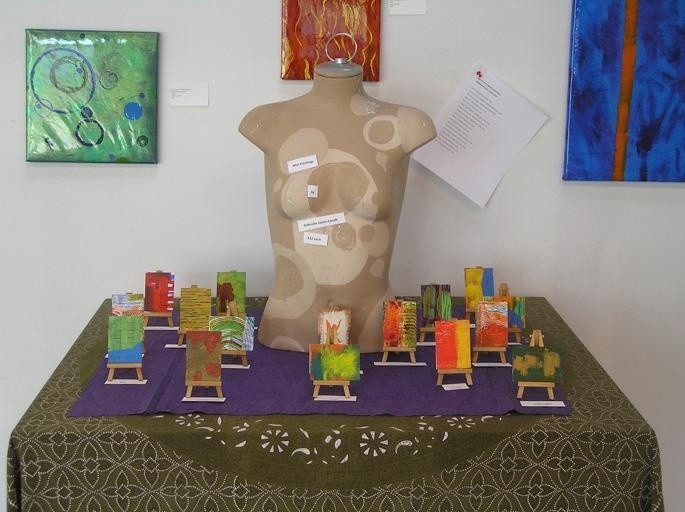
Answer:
[235, 57, 441, 356]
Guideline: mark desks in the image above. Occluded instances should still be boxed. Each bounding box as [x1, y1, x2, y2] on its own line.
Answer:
[11, 296, 655, 510]
